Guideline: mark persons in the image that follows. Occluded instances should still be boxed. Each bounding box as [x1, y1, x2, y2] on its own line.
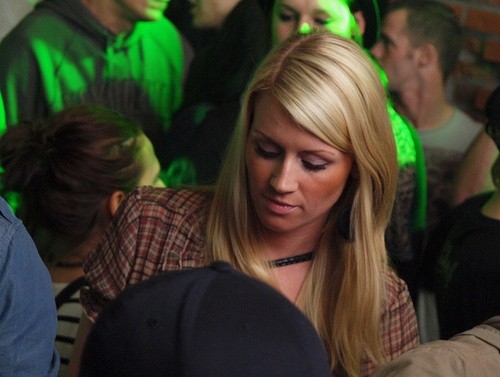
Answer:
[0, 105, 167, 377]
[0, 0, 195, 216]
[80, 262, 332, 377]
[272, 0, 427, 318]
[66, 28, 422, 377]
[161, 0, 273, 189]
[0, 198, 62, 377]
[368, 1, 500, 377]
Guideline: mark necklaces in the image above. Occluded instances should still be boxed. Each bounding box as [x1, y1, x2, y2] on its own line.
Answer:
[270, 253, 313, 267]
[56, 261, 83, 269]
